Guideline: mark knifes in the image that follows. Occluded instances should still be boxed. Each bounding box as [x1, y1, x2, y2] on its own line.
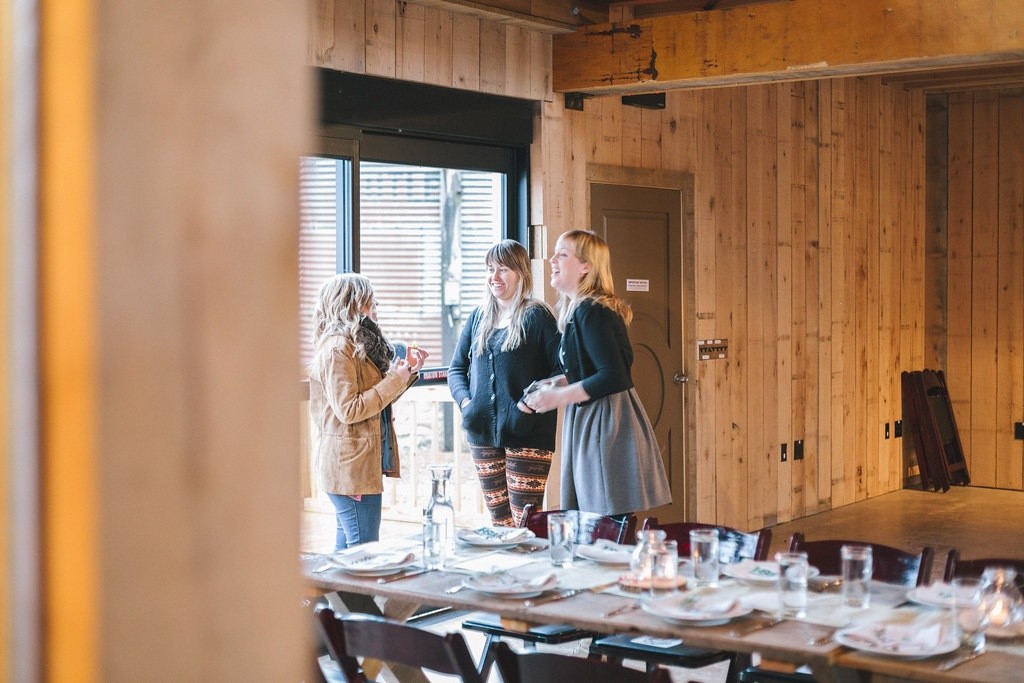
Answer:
[938, 654, 981, 671]
[524, 590, 583, 606]
[731, 619, 785, 636]
[377, 569, 434, 584]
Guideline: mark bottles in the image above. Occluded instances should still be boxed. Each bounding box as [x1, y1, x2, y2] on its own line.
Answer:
[422, 467, 457, 564]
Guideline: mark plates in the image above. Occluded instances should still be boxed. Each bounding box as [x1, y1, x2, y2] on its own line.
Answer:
[460, 527, 538, 550]
[724, 560, 822, 587]
[574, 547, 627, 567]
[330, 548, 416, 576]
[642, 591, 755, 628]
[464, 572, 557, 600]
[906, 587, 974, 610]
[836, 625, 960, 660]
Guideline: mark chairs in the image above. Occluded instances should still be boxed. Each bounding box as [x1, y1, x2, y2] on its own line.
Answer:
[306, 504, 1024, 683]
[900, 367, 973, 494]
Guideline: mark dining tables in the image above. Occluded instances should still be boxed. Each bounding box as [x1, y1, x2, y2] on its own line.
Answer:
[307, 533, 1024, 683]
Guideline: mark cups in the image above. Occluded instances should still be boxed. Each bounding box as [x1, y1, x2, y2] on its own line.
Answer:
[949, 580, 987, 654]
[841, 546, 873, 610]
[549, 512, 576, 571]
[688, 529, 720, 587]
[648, 541, 678, 607]
[776, 551, 809, 619]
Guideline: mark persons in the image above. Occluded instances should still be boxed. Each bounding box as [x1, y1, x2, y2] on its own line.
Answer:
[446, 239, 564, 528]
[524, 230, 674, 519]
[310, 272, 429, 553]
